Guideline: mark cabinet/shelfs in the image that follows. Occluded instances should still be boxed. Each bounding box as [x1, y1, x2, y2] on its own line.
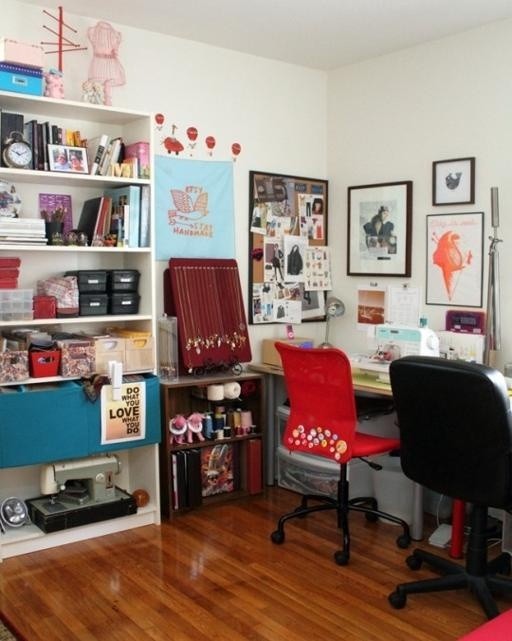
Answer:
[0, 90, 163, 559]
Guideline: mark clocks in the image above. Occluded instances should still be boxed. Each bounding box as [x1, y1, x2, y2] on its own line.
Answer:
[3, 131, 33, 169]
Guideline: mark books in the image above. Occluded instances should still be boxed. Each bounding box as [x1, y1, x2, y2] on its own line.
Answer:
[0, 110, 150, 248]
[1, 327, 53, 352]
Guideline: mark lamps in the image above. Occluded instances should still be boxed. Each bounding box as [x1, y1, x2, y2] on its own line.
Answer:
[317, 296, 345, 349]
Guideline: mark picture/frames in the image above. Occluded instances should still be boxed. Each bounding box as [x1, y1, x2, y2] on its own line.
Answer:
[432, 156, 475, 207]
[347, 180, 413, 278]
[425, 212, 485, 308]
[47, 143, 89, 175]
[248, 170, 329, 325]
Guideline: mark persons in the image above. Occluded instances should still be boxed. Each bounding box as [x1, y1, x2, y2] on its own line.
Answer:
[270, 243, 283, 281]
[288, 244, 304, 275]
[369, 205, 389, 232]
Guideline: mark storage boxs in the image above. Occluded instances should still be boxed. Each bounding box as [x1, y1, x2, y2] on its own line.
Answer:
[0, 61, 43, 96]
[0, 267, 162, 468]
[276, 405, 400, 505]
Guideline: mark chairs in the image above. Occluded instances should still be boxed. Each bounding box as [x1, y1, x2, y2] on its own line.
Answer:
[270, 343, 408, 566]
[388, 355, 512, 620]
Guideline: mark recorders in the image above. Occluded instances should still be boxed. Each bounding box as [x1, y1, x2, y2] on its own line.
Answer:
[446, 310, 486, 334]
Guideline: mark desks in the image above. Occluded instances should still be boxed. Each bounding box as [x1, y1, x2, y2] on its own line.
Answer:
[159, 371, 266, 523]
[248, 363, 512, 555]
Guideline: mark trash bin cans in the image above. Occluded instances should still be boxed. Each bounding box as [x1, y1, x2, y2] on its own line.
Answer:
[371, 456, 415, 527]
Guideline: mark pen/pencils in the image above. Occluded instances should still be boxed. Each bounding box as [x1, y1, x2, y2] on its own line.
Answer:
[40, 205, 67, 223]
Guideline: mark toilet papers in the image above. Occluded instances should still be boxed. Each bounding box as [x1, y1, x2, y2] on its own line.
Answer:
[224, 381, 242, 400]
[207, 384, 224, 402]
